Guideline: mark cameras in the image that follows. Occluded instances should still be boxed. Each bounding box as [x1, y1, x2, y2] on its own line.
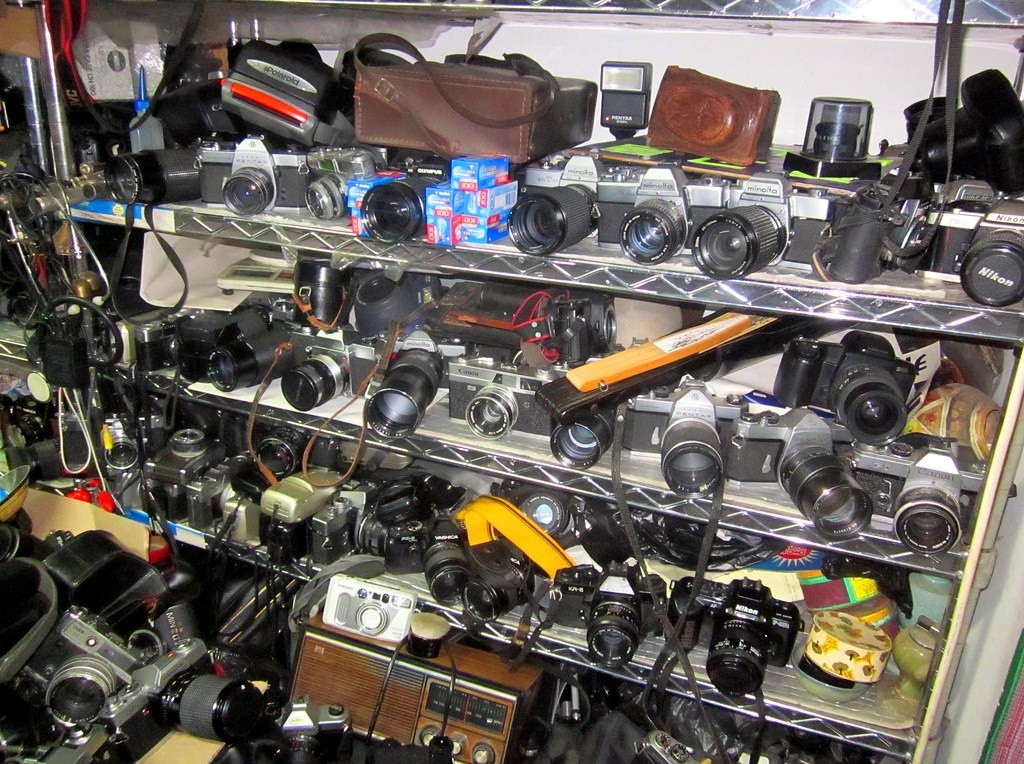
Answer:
[0, 38, 1024, 764]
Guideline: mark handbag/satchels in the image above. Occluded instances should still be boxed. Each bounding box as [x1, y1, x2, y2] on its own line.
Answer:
[353, 32, 559, 165]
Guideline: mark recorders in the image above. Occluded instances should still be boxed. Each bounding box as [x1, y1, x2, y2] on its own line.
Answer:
[283, 611, 550, 764]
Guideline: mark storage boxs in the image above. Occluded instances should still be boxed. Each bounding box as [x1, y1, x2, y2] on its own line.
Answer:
[346, 153, 519, 246]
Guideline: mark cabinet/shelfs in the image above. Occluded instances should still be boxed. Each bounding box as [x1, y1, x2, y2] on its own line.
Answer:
[0, 2, 1024, 764]
